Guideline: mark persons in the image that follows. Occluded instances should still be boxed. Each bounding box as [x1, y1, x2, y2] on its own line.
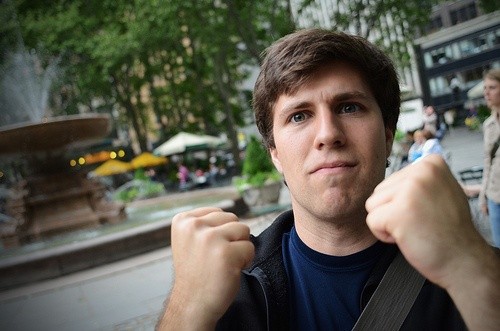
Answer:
[394, 76, 467, 172]
[155, 31, 500, 331]
[480, 72, 500, 249]
[177, 160, 219, 190]
